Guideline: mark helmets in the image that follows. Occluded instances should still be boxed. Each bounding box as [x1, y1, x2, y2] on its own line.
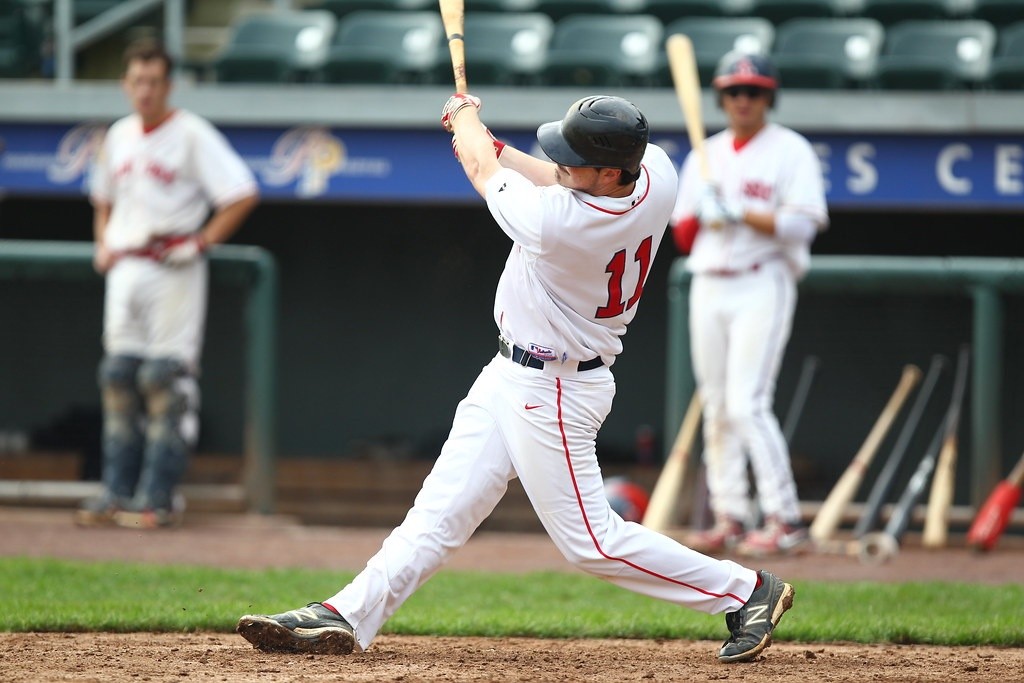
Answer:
[536, 96, 650, 175]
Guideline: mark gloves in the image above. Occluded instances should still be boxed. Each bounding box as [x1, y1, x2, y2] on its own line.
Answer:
[452, 125, 505, 162]
[441, 94, 481, 132]
[155, 235, 204, 267]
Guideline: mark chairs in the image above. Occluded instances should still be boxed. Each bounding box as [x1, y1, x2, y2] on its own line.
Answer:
[0, 2, 1024, 91]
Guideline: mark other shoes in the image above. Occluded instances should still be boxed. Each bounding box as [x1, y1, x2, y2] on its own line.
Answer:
[694, 518, 743, 552]
[77, 504, 182, 529]
[739, 519, 796, 550]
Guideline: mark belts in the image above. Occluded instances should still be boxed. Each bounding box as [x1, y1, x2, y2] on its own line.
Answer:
[499, 335, 605, 372]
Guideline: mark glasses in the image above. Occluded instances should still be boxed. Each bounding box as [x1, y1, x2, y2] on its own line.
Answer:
[725, 85, 762, 99]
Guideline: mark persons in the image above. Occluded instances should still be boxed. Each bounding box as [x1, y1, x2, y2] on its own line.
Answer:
[71, 57, 259, 532]
[666, 47, 828, 558]
[234, 101, 796, 663]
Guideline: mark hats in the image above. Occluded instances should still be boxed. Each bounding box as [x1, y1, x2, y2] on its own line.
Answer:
[713, 49, 776, 89]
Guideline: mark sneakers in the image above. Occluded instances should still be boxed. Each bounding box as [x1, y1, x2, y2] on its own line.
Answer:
[237, 602, 356, 655]
[718, 570, 794, 663]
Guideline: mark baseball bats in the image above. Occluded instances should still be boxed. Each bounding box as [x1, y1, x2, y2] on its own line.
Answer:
[638, 342, 1024, 551]
[438, 0, 468, 98]
[666, 32, 724, 233]
